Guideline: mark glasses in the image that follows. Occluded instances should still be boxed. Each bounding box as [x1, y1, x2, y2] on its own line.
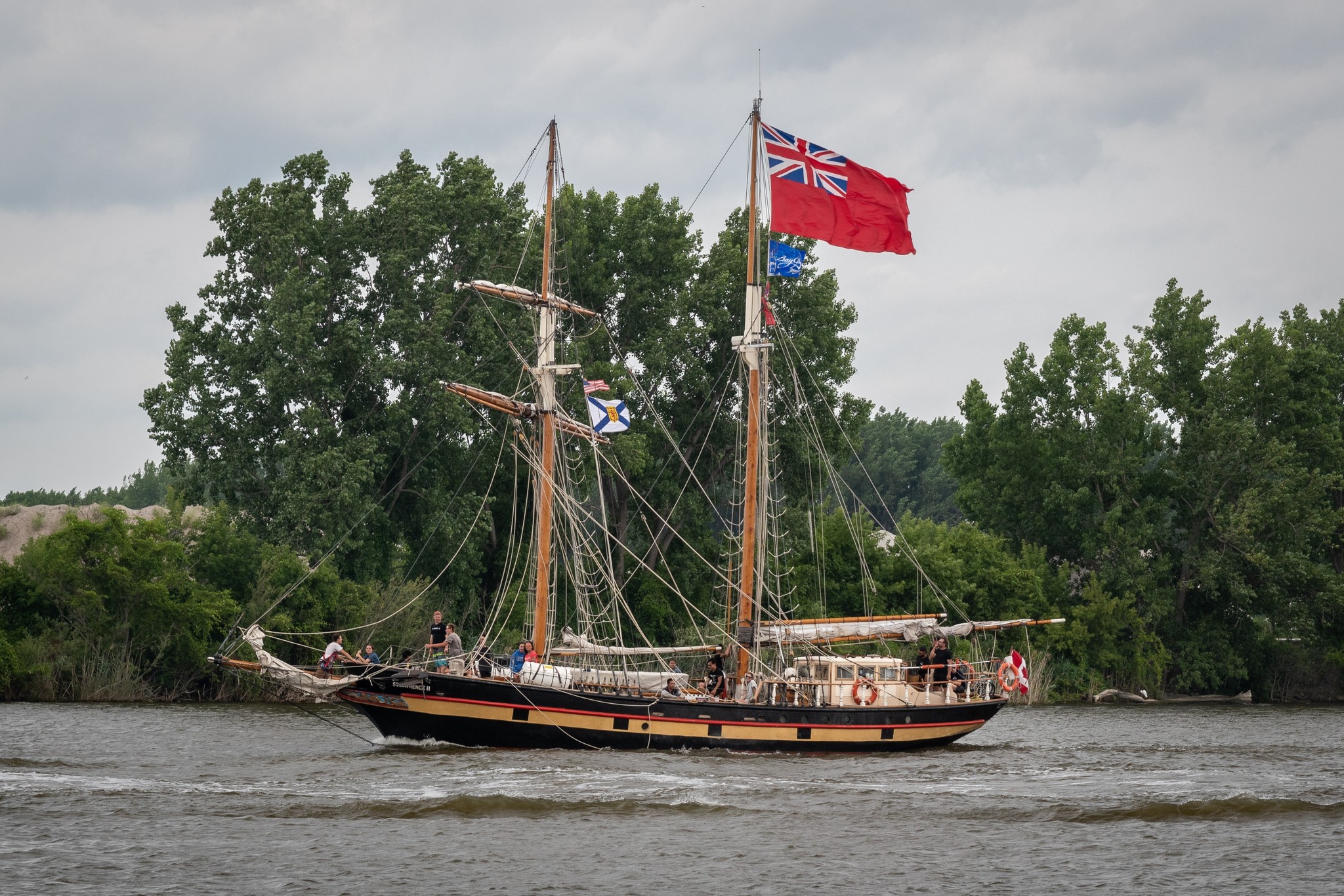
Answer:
[446, 628, 449, 630]
[746, 675, 752, 679]
[951, 666, 956, 669]
[938, 641, 942, 643]
[918, 651, 924, 654]
[708, 663, 713, 667]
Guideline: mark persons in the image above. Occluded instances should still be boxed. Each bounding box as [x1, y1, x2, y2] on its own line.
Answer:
[471, 633, 494, 679]
[668, 659, 682, 673]
[697, 657, 725, 702]
[914, 646, 932, 694]
[930, 638, 953, 694]
[712, 643, 733, 671]
[314, 633, 361, 679]
[356, 644, 380, 665]
[523, 641, 538, 663]
[395, 650, 429, 672]
[928, 636, 940, 654]
[429, 611, 449, 673]
[510, 642, 526, 682]
[425, 624, 465, 677]
[736, 672, 758, 704]
[660, 678, 685, 699]
[949, 662, 966, 693]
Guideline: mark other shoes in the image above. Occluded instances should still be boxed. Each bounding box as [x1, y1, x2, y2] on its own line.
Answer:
[697, 687, 708, 692]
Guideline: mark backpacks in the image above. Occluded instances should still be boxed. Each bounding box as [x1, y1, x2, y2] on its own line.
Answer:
[320, 652, 338, 672]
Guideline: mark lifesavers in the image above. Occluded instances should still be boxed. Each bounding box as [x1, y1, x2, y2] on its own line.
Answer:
[952, 660, 976, 686]
[852, 677, 878, 707]
[997, 662, 1020, 692]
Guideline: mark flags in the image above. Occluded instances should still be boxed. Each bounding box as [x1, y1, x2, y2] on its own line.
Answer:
[758, 121, 917, 257]
[1010, 651, 1029, 695]
[768, 239, 806, 278]
[586, 395, 631, 433]
[582, 380, 609, 395]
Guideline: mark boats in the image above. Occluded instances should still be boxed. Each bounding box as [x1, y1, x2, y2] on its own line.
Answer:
[203, 93, 1029, 753]
